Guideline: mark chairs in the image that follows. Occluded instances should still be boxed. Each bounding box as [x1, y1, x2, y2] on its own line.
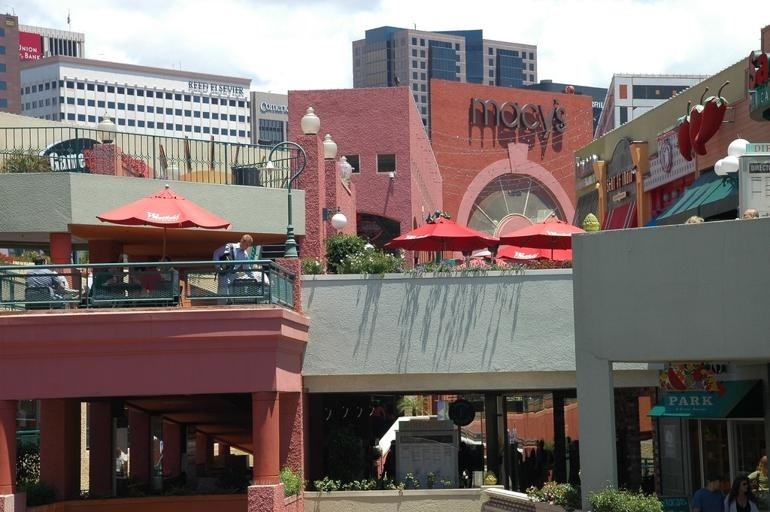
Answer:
[231, 278, 258, 304]
[153, 279, 173, 307]
[23, 285, 52, 308]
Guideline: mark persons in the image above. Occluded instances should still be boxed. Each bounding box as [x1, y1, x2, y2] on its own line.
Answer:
[25, 253, 180, 309]
[742, 208, 760, 220]
[213, 234, 257, 306]
[690, 472, 727, 511]
[685, 215, 704, 224]
[380, 439, 396, 481]
[723, 475, 760, 511]
[458, 435, 581, 492]
[746, 455, 770, 508]
[237, 263, 270, 286]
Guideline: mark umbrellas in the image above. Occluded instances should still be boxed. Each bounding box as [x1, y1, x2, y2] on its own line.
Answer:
[382, 215, 500, 261]
[94, 185, 231, 258]
[498, 213, 589, 260]
[495, 246, 573, 263]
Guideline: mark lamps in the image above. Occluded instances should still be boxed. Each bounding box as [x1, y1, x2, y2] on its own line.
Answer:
[323, 206, 347, 230]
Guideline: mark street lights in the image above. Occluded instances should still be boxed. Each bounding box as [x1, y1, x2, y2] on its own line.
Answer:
[265, 141, 308, 259]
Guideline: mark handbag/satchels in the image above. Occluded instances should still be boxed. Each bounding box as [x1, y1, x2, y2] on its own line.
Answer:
[218, 256, 235, 274]
